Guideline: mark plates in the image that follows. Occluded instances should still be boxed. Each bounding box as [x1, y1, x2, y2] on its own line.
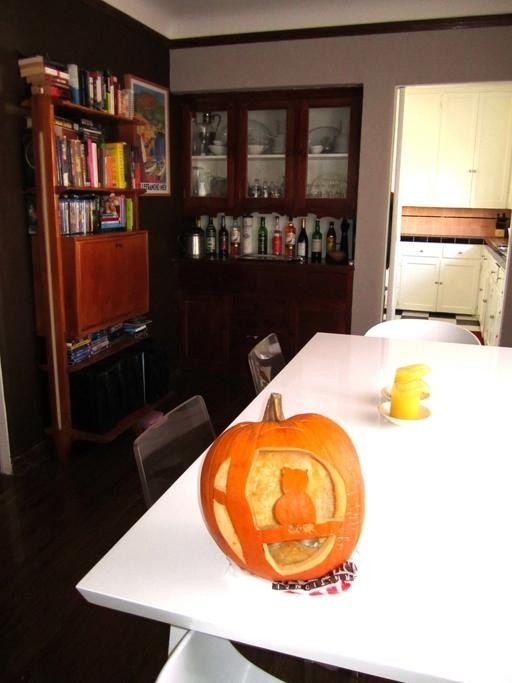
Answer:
[381, 384, 431, 401]
[380, 402, 430, 426]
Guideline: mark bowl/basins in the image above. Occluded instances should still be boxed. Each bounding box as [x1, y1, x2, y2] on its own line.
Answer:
[208, 140, 266, 155]
[310, 145, 323, 154]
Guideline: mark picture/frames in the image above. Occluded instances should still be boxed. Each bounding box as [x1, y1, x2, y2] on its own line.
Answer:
[124, 73, 171, 197]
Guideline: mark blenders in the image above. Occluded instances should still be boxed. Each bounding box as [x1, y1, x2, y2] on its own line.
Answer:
[192, 110, 222, 157]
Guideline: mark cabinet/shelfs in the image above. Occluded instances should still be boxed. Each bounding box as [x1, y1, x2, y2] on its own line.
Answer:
[395, 79, 512, 210]
[168, 85, 362, 220]
[477, 245, 507, 346]
[21, 94, 162, 473]
[169, 258, 353, 385]
[391, 241, 483, 316]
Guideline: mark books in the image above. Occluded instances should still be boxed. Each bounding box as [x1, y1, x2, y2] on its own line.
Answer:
[53, 116, 136, 188]
[65, 314, 152, 363]
[58, 191, 134, 235]
[20, 55, 134, 118]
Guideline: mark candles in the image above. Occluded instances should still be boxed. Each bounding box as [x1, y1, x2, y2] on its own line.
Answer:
[390, 363, 427, 419]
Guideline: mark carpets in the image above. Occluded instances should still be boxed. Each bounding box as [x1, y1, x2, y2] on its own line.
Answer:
[383, 309, 481, 332]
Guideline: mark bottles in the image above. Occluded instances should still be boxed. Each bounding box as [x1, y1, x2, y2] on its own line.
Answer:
[191, 215, 350, 264]
[193, 166, 226, 198]
[252, 175, 285, 198]
[495, 211, 506, 229]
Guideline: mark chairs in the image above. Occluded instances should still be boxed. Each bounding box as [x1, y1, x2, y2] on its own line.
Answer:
[134, 395, 217, 509]
[247, 332, 286, 394]
[362, 318, 480, 345]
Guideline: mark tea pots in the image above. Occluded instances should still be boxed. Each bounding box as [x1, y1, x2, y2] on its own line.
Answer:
[272, 135, 286, 153]
[320, 136, 337, 154]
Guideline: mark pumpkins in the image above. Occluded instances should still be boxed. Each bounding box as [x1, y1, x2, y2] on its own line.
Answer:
[197, 393, 365, 582]
